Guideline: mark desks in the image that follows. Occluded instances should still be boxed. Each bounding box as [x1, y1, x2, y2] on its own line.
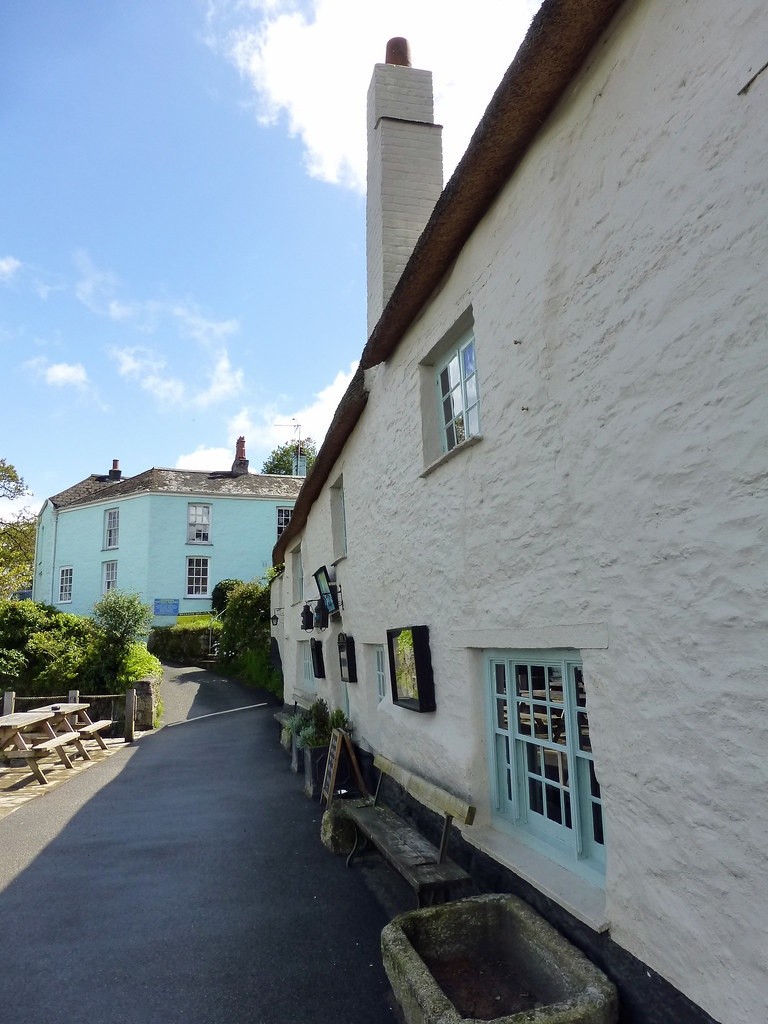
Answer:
[27, 703, 109, 760]
[0, 713, 74, 785]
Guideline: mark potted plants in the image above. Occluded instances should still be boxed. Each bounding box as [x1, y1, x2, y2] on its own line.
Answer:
[298, 710, 348, 799]
[281, 698, 330, 774]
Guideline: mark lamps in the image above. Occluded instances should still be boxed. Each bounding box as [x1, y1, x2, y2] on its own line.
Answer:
[301, 599, 318, 633]
[314, 599, 328, 632]
[270, 608, 284, 626]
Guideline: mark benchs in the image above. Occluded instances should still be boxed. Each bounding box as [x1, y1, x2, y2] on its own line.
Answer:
[342, 755, 476, 908]
[78, 720, 112, 735]
[32, 732, 80, 751]
